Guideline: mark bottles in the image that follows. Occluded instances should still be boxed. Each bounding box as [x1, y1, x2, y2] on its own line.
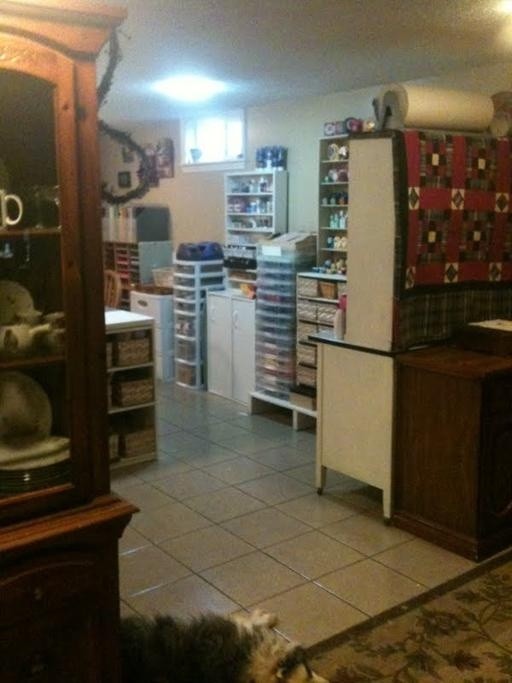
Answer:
[332, 292, 347, 340]
[322, 190, 347, 228]
[245, 177, 272, 226]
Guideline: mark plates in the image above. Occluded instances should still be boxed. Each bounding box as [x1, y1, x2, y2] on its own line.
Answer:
[0, 370, 55, 454]
[0, 436, 72, 497]
[0, 279, 35, 325]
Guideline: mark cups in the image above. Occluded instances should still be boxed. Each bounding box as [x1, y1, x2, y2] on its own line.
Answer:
[22, 183, 63, 230]
[1, 186, 25, 232]
[43, 310, 66, 351]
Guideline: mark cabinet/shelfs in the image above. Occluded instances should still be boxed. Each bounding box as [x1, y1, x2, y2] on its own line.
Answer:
[105, 128, 394, 525]
[0, 0, 146, 683]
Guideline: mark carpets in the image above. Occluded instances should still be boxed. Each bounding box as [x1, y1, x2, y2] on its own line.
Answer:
[279, 545, 512, 683]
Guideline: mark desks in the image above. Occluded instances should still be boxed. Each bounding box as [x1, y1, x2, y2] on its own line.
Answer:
[392, 343, 511, 559]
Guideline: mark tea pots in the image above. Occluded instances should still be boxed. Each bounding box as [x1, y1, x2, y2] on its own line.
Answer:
[1, 321, 50, 358]
